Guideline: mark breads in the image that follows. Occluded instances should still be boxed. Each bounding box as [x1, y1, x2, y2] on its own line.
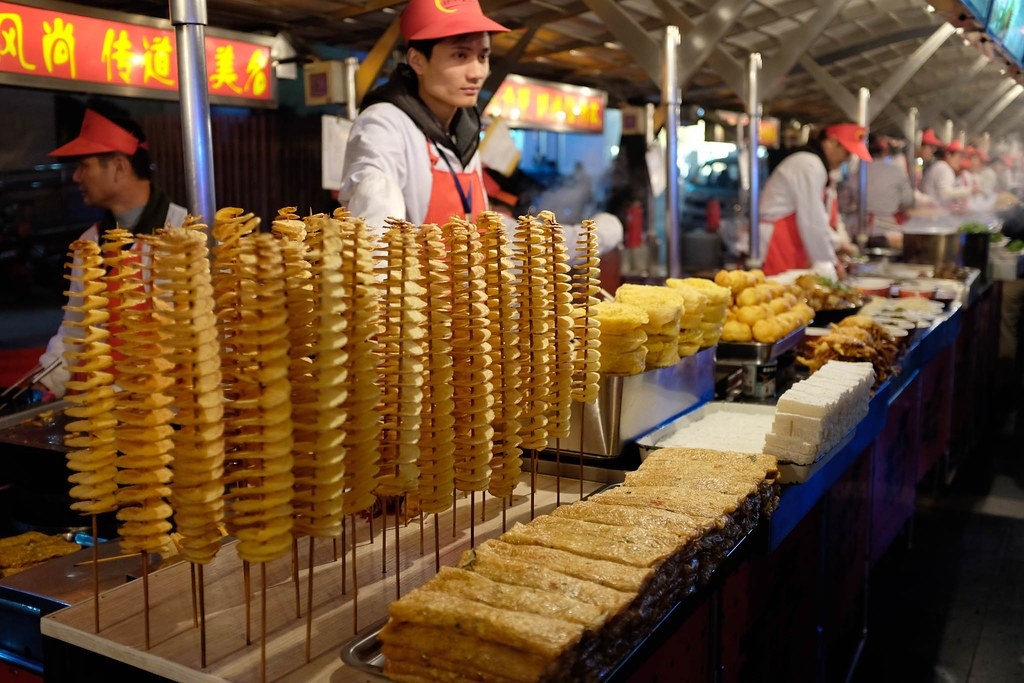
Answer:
[715, 267, 813, 343]
[595, 278, 732, 375]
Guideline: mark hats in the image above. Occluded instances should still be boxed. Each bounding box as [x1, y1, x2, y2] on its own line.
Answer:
[47, 106, 146, 156]
[400, 1, 511, 44]
[823, 123, 872, 161]
[944, 139, 966, 152]
[921, 128, 943, 145]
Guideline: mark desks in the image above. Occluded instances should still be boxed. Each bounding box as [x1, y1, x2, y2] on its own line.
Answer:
[0, 267, 982, 683]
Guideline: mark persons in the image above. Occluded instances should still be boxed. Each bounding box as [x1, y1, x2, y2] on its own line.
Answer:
[26, 100, 188, 402]
[335, 0, 493, 314]
[536, 150, 744, 283]
[756, 120, 867, 274]
[861, 134, 914, 236]
[887, 129, 1024, 216]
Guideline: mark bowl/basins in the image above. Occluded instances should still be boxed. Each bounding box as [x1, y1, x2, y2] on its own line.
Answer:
[897, 287, 935, 299]
[842, 276, 892, 297]
